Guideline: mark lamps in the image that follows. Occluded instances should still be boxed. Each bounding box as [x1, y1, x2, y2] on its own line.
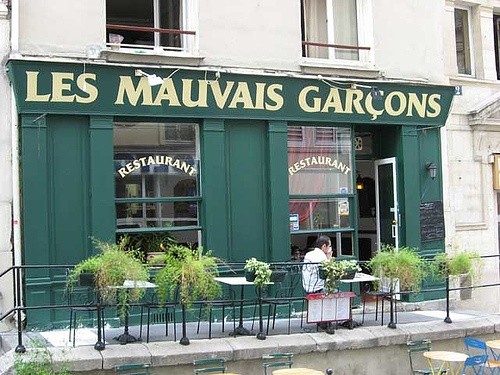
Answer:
[135, 70, 165, 86]
[357, 170, 363, 189]
[426, 161, 437, 180]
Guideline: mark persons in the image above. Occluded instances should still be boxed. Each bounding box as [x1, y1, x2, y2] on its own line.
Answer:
[301, 234, 359, 329]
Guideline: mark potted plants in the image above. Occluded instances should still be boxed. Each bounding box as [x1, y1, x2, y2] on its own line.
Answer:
[318, 259, 360, 296]
[244, 258, 273, 293]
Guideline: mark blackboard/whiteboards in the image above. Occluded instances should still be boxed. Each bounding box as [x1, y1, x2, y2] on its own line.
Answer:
[419, 200, 445, 242]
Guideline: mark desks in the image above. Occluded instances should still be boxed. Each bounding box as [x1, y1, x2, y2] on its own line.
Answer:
[215, 277, 274, 337]
[337, 272, 380, 331]
[272, 368, 326, 375]
[485, 340, 500, 375]
[424, 351, 470, 375]
[100, 280, 160, 345]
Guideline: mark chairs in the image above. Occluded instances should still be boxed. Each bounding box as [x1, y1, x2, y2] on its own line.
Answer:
[407, 337, 489, 375]
[64, 267, 322, 347]
[261, 352, 294, 375]
[194, 358, 225, 375]
